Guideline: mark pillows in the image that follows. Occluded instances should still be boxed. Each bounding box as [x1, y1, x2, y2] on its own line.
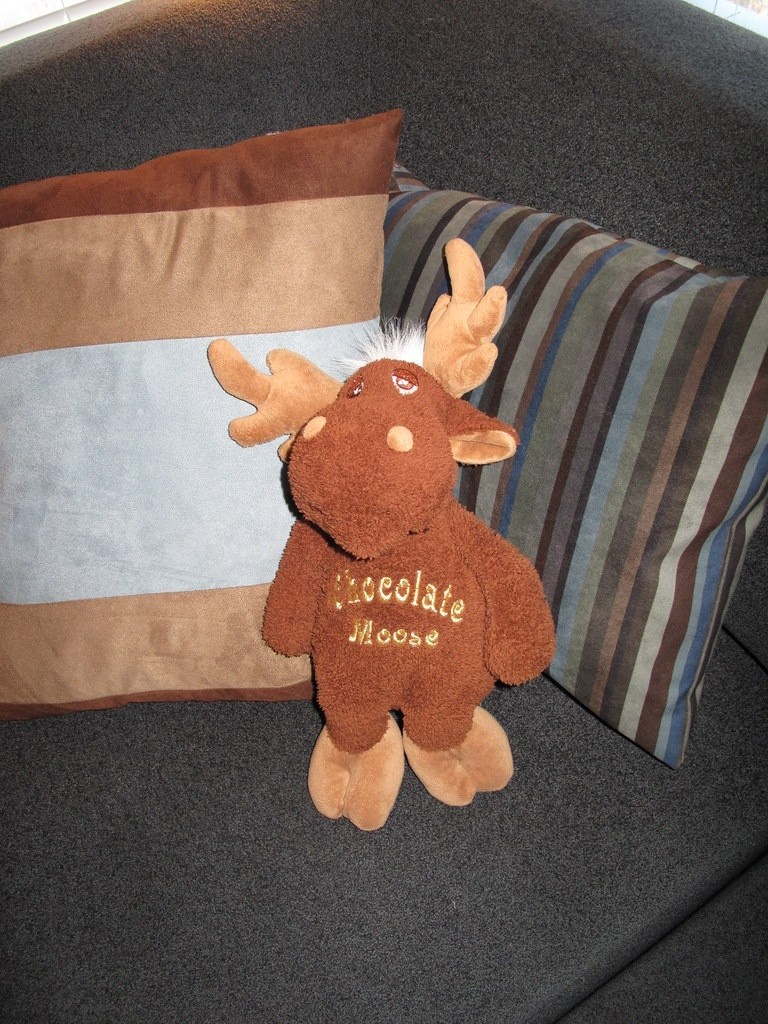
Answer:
[381, 162, 767, 772]
[0, 108, 406, 724]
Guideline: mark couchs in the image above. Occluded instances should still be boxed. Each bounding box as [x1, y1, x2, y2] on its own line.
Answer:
[0, 0, 768, 1024]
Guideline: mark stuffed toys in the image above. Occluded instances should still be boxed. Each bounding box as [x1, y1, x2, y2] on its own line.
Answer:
[207, 240, 555, 832]
